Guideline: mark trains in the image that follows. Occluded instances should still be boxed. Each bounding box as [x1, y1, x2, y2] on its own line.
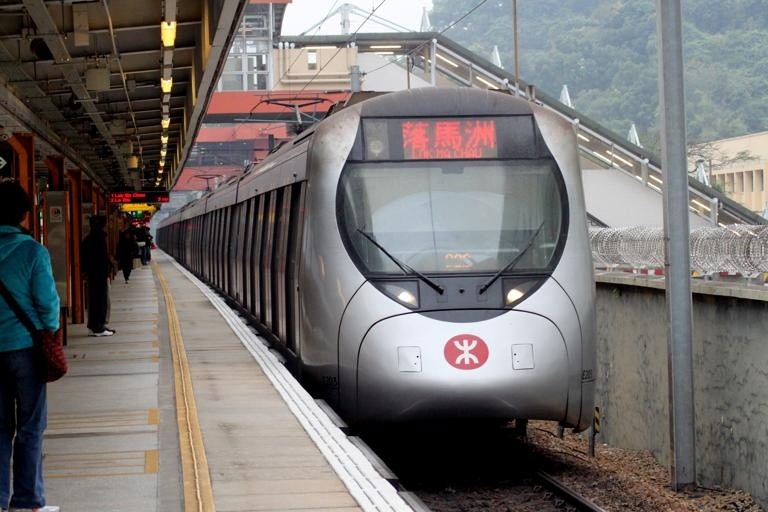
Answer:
[155, 86, 599, 436]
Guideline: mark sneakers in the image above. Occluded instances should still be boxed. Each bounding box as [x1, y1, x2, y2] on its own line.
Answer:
[9, 505, 59, 512]
[87, 326, 115, 337]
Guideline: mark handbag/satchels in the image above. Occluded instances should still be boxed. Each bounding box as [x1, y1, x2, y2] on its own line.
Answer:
[32, 329, 69, 382]
[108, 257, 118, 280]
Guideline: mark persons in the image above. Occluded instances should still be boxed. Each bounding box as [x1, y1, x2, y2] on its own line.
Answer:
[0, 181, 60, 512]
[81, 214, 153, 337]
